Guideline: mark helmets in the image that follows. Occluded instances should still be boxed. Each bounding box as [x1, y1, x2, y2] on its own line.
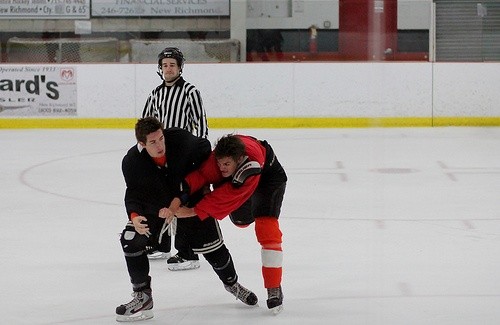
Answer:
[158, 47, 185, 66]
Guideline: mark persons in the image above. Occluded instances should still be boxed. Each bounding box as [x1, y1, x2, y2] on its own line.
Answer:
[116, 117, 258, 321]
[143, 47, 208, 138]
[159, 135, 288, 312]
[0, 29, 288, 64]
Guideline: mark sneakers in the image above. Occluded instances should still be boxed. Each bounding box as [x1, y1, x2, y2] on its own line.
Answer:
[267, 285, 284, 313]
[146, 246, 172, 260]
[167, 252, 200, 271]
[116, 287, 154, 321]
[224, 281, 259, 307]
